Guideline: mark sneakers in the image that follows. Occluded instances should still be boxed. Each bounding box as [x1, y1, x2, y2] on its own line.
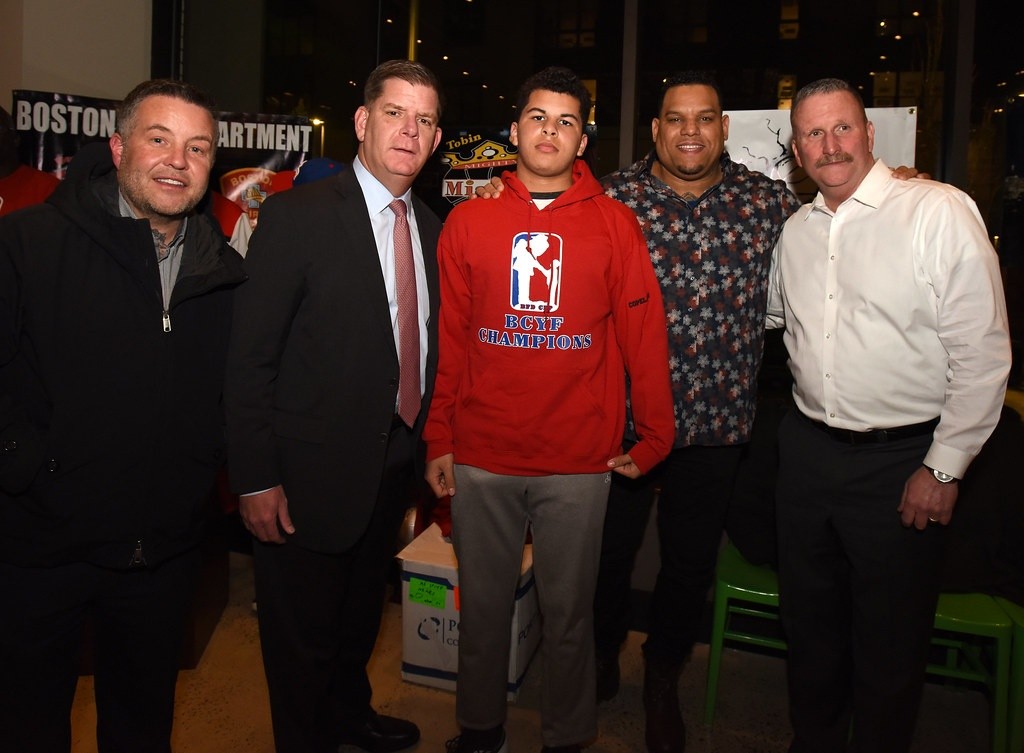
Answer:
[446, 726, 508, 753]
[541, 745, 581, 753]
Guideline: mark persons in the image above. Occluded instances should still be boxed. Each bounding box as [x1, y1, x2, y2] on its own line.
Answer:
[473, 73, 931, 752]
[764, 74, 1014, 750]
[239, 56, 442, 752]
[423, 67, 677, 753]
[0, 77, 244, 753]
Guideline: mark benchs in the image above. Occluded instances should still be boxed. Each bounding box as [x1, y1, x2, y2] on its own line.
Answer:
[702, 539, 1024, 753]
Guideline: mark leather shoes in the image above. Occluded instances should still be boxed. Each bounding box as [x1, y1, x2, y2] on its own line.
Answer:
[597, 661, 620, 701]
[643, 670, 685, 753]
[339, 714, 421, 753]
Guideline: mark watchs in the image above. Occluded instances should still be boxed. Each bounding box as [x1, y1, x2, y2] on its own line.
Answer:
[927, 466, 957, 484]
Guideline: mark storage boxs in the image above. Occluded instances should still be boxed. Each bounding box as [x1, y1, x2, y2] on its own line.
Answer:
[393, 521, 542, 702]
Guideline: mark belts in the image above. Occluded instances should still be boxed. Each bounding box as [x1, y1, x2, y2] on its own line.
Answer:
[792, 399, 941, 444]
[391, 412, 405, 428]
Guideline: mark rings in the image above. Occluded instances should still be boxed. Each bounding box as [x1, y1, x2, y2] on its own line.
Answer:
[930, 516, 939, 522]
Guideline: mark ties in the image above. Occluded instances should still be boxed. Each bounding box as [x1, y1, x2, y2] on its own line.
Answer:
[388, 198, 421, 429]
